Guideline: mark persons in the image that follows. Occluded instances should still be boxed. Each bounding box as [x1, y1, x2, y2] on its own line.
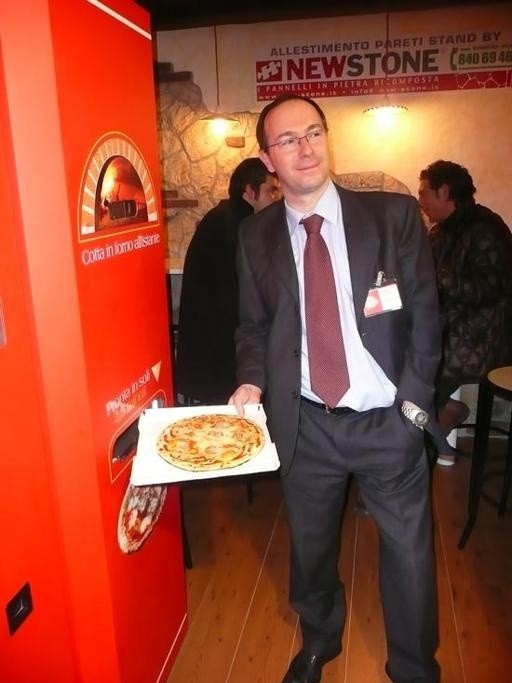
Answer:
[418, 159, 511, 469]
[227, 91, 445, 681]
[174, 156, 283, 403]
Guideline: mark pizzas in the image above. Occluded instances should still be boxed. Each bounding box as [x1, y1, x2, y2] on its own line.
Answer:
[156, 413, 265, 472]
[117, 483, 167, 554]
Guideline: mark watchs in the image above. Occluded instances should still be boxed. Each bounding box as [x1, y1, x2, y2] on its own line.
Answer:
[401, 406, 429, 428]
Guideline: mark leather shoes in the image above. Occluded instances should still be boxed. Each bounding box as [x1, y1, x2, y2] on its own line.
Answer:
[282, 641, 342, 683]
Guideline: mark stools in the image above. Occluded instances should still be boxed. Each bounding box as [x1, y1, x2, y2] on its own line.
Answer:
[458, 366, 511, 553]
[174, 372, 254, 568]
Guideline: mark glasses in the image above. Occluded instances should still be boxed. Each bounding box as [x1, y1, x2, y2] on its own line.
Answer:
[265, 128, 326, 152]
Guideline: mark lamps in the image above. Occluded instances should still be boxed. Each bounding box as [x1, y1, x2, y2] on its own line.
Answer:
[363, 0, 408, 126]
[196, 0, 239, 139]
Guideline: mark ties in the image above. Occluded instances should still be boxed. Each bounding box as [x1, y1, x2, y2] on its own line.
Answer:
[299, 213, 351, 410]
